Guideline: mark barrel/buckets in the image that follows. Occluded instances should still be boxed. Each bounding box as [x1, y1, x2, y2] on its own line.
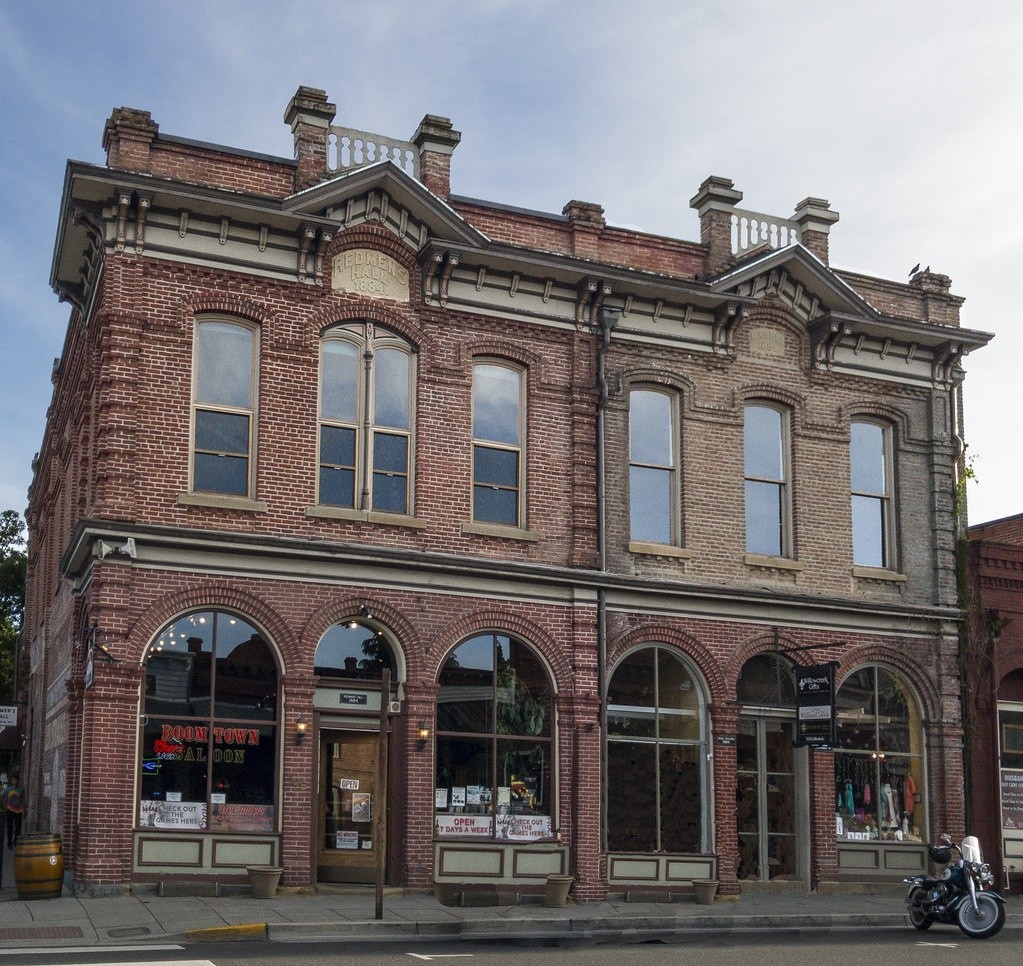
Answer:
[692, 879, 720, 905]
[14, 834, 64, 901]
[543, 875, 574, 908]
[246, 865, 283, 898]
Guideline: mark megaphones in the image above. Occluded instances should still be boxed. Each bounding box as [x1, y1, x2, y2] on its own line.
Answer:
[97, 540, 116, 560]
[117, 538, 137, 558]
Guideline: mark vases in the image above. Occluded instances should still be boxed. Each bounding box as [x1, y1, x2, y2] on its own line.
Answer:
[246, 865, 284, 900]
[691, 879, 719, 905]
[542, 875, 574, 907]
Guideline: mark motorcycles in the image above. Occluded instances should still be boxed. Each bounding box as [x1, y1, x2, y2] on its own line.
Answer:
[903, 833, 1007, 940]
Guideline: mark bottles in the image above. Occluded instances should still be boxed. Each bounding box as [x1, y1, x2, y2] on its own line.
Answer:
[903, 812, 910, 834]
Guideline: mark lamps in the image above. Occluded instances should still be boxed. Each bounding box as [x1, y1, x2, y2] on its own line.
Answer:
[415, 716, 429, 751]
[360, 604, 373, 619]
[679, 680, 691, 691]
[103, 642, 110, 651]
[640, 686, 650, 696]
[295, 711, 307, 746]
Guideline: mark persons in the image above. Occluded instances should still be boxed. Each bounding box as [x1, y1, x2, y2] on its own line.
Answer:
[0, 774, 28, 851]
[879, 784, 901, 832]
